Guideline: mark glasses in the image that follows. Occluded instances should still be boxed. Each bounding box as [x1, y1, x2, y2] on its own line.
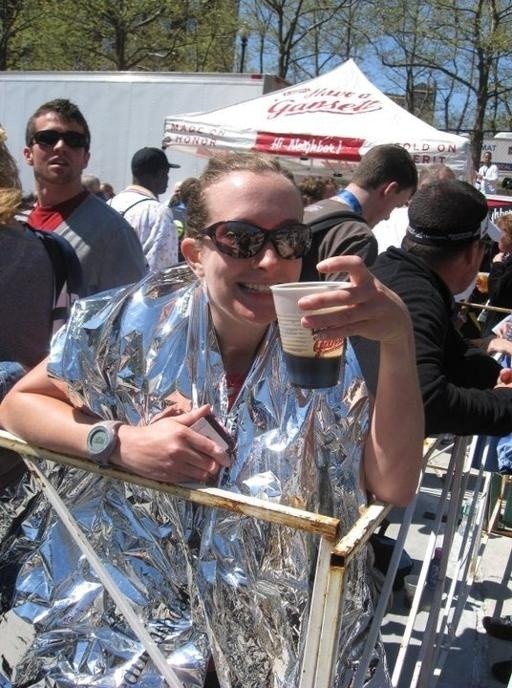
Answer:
[195, 220, 312, 258]
[28, 130, 89, 149]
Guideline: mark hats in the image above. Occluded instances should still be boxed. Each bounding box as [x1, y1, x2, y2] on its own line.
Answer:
[131, 146, 181, 175]
[404, 211, 502, 246]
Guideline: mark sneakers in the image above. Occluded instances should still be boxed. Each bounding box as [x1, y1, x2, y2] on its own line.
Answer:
[483, 616, 512, 639]
[492, 659, 512, 684]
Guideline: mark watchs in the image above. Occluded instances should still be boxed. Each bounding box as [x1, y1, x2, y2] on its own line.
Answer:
[86, 417, 121, 467]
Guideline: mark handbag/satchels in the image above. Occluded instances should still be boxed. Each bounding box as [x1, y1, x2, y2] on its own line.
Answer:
[35, 226, 71, 337]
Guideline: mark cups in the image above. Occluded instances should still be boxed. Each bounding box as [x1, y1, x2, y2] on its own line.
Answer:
[271, 281, 353, 387]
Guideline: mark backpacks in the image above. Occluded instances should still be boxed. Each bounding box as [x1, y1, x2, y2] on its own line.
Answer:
[33, 223, 85, 352]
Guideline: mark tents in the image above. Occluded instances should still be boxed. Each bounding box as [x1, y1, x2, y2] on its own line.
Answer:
[162, 58, 469, 171]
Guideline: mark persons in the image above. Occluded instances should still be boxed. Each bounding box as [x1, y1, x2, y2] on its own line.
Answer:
[24, 100, 147, 294]
[0, 151, 424, 680]
[483, 211, 511, 336]
[1, 138, 59, 489]
[474, 152, 498, 195]
[165, 176, 198, 224]
[102, 147, 179, 272]
[299, 142, 419, 284]
[348, 152, 512, 435]
